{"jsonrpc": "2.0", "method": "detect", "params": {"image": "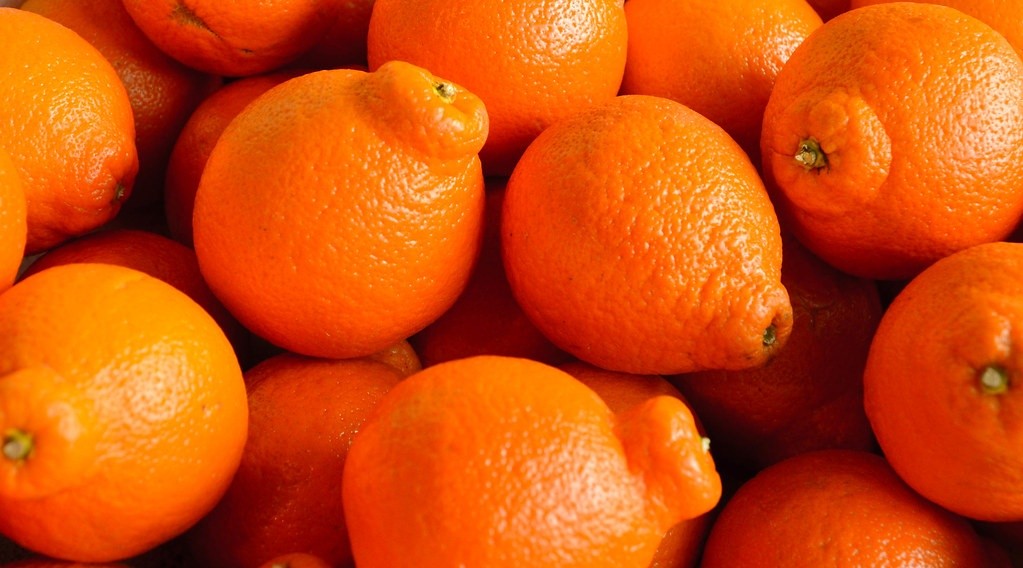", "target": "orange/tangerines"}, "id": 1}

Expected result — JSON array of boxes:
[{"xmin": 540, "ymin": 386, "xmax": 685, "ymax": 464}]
[{"xmin": 0, "ymin": 0, "xmax": 1023, "ymax": 568}]
